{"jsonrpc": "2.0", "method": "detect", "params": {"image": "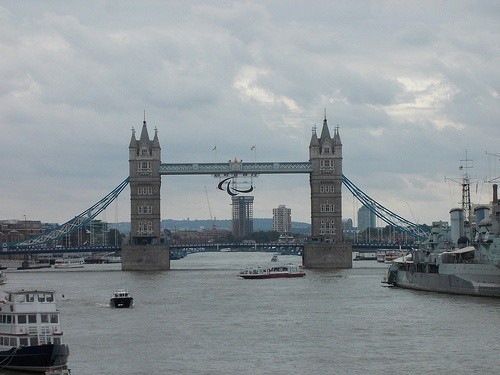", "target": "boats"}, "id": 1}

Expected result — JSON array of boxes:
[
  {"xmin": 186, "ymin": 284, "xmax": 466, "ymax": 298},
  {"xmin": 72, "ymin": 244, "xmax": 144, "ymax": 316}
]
[
  {"xmin": 55, "ymin": 257, "xmax": 85, "ymax": 268},
  {"xmin": 271, "ymin": 256, "xmax": 278, "ymax": 262},
  {"xmin": 382, "ymin": 204, "xmax": 500, "ymax": 298},
  {"xmin": 109, "ymin": 292, "xmax": 133, "ymax": 308},
  {"xmin": 237, "ymin": 265, "xmax": 306, "ymax": 280},
  {"xmin": 0, "ymin": 289, "xmax": 70, "ymax": 370}
]
